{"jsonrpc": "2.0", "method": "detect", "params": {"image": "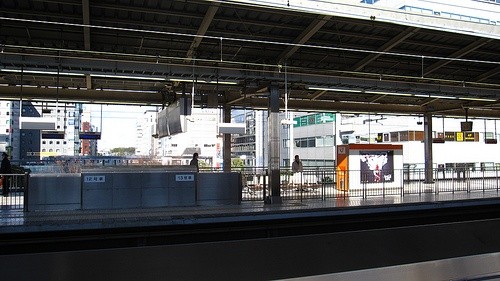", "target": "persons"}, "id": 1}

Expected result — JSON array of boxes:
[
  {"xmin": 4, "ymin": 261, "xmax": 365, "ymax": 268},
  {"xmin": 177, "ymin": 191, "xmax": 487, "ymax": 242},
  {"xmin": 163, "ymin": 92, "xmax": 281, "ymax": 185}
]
[
  {"xmin": 292, "ymin": 154, "xmax": 305, "ymax": 191},
  {"xmin": 190, "ymin": 152, "xmax": 199, "ymax": 172},
  {"xmin": 0, "ymin": 152, "xmax": 12, "ymax": 197}
]
